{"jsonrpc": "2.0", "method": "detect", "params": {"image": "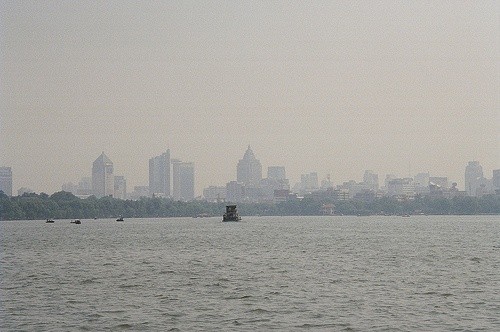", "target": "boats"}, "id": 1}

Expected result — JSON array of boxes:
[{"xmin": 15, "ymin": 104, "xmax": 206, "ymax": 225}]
[
  {"xmin": 46, "ymin": 218, "xmax": 54, "ymax": 223},
  {"xmin": 222, "ymin": 205, "xmax": 242, "ymax": 223},
  {"xmin": 70, "ymin": 219, "xmax": 81, "ymax": 224},
  {"xmin": 116, "ymin": 217, "xmax": 124, "ymax": 221}
]
